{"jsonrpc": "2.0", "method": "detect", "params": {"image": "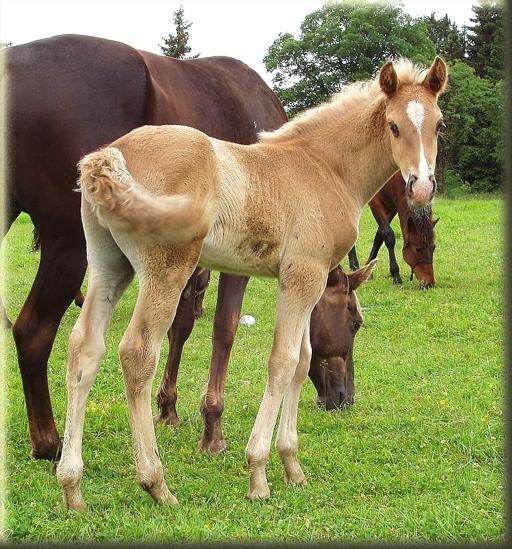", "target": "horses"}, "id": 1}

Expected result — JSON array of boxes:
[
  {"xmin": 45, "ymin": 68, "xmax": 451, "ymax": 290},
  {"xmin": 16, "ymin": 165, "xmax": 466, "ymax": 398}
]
[
  {"xmin": 31, "ymin": 226, "xmax": 211, "ymax": 320},
  {"xmin": 348, "ymin": 170, "xmax": 440, "ymax": 289},
  {"xmin": 56, "ymin": 56, "xmax": 448, "ymax": 514},
  {"xmin": 0, "ymin": 34, "xmax": 376, "ymax": 462}
]
